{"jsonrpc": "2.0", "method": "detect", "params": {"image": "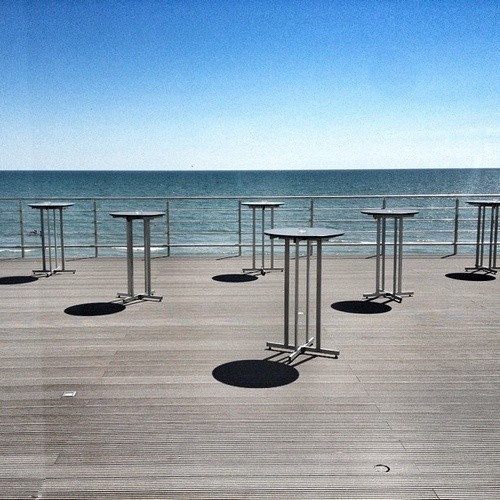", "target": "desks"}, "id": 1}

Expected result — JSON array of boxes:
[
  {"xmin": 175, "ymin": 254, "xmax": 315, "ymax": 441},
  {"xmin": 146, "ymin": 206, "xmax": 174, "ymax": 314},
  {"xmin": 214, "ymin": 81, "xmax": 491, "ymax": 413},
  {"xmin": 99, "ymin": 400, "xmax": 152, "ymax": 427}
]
[
  {"xmin": 465, "ymin": 200, "xmax": 500, "ymax": 275},
  {"xmin": 27, "ymin": 202, "xmax": 76, "ymax": 278},
  {"xmin": 262, "ymin": 227, "xmax": 340, "ymax": 363},
  {"xmin": 110, "ymin": 212, "xmax": 166, "ymax": 304},
  {"xmin": 361, "ymin": 209, "xmax": 420, "ymax": 303},
  {"xmin": 241, "ymin": 202, "xmax": 284, "ymax": 275}
]
